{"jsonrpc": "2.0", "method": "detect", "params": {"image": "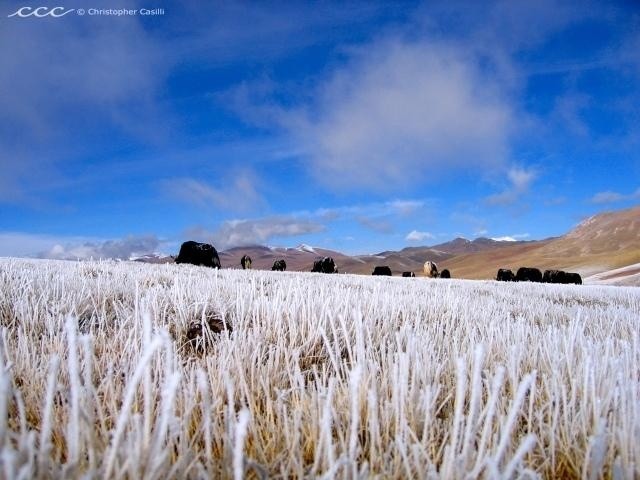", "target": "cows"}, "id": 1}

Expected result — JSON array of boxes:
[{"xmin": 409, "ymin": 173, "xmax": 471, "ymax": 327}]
[
  {"xmin": 440, "ymin": 269, "xmax": 451, "ymax": 279},
  {"xmin": 402, "ymin": 271, "xmax": 415, "ymax": 277},
  {"xmin": 424, "ymin": 261, "xmax": 440, "ymax": 278},
  {"xmin": 311, "ymin": 256, "xmax": 339, "ymax": 274},
  {"xmin": 241, "ymin": 255, "xmax": 254, "ymax": 269},
  {"xmin": 493, "ymin": 267, "xmax": 582, "ymax": 285},
  {"xmin": 372, "ymin": 266, "xmax": 392, "ymax": 276},
  {"xmin": 170, "ymin": 240, "xmax": 222, "ymax": 269},
  {"xmin": 270, "ymin": 260, "xmax": 286, "ymax": 271}
]
